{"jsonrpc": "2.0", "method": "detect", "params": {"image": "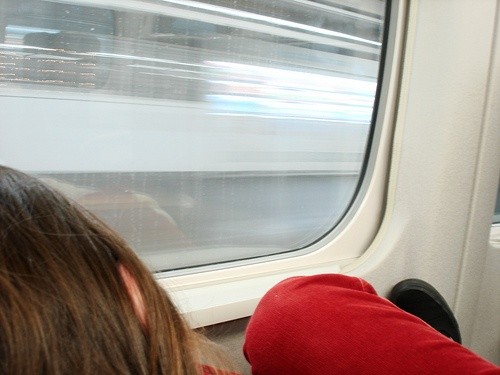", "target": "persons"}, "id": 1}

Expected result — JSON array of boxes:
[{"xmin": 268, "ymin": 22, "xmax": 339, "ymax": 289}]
[{"xmin": 0, "ymin": 166, "xmax": 500, "ymax": 375}]
